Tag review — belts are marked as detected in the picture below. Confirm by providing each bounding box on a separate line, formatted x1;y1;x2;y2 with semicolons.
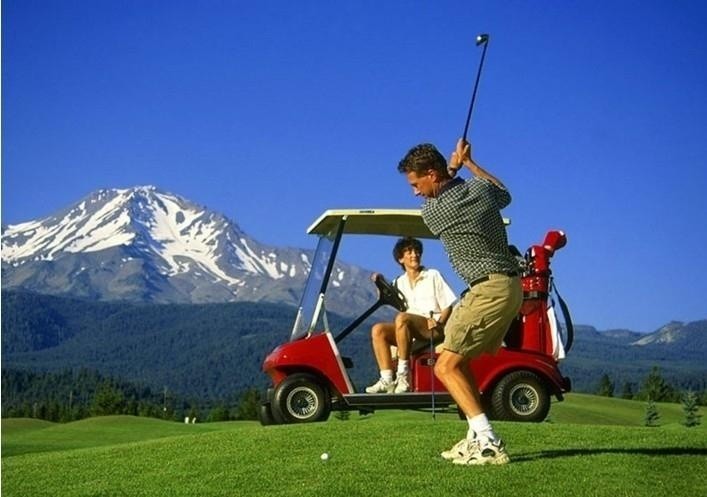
468;272;518;288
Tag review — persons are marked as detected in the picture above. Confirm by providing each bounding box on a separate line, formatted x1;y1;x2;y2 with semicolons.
364;235;458;394
396;136;523;465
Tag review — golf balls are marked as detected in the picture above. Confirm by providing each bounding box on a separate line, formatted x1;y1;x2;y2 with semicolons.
321;453;329;460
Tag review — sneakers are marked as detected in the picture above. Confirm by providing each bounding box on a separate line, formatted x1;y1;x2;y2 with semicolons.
441;438;469;460
393;371;413;394
453;438;510;466
366;377;393;394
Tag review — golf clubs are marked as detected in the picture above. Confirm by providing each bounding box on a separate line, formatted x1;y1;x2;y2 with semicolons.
462;34;489;142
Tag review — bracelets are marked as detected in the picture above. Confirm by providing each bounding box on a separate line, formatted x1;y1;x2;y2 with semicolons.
449;167;458;174
